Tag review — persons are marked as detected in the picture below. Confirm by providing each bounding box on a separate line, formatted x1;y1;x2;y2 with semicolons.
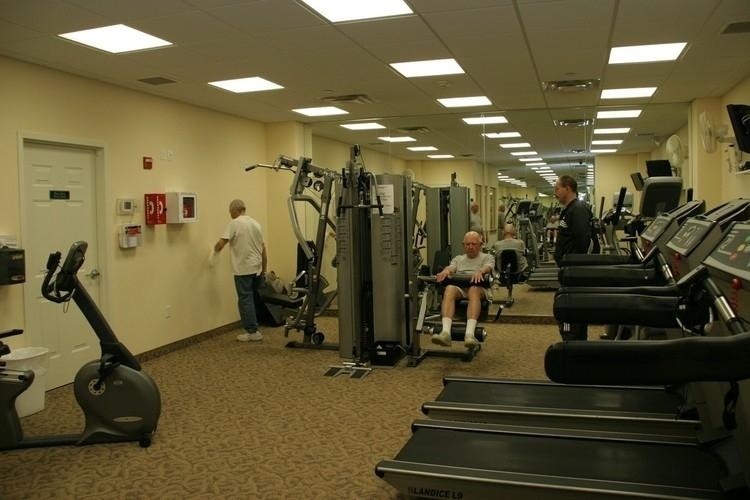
546;214;559;243
496;223;528;274
498;204;505;240
431;231;496;347
554;176;593;340
470;202;487;246
214;199;267;342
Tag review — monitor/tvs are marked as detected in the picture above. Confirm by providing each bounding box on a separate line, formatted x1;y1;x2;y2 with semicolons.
645;159;673;177
726;104;750;153
629;172;643;190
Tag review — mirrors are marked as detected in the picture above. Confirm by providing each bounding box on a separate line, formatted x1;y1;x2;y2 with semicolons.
310;102;692;317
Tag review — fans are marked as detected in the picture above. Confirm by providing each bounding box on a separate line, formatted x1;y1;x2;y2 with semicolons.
665;134;688;168
700;110;744;179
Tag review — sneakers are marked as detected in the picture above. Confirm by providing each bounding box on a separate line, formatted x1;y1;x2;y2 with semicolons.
431;330;451;346
464;334;480;348
236;331;263;342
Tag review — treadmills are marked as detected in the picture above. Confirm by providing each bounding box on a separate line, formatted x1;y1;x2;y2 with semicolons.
528;208;633;289
374;200;749;499
